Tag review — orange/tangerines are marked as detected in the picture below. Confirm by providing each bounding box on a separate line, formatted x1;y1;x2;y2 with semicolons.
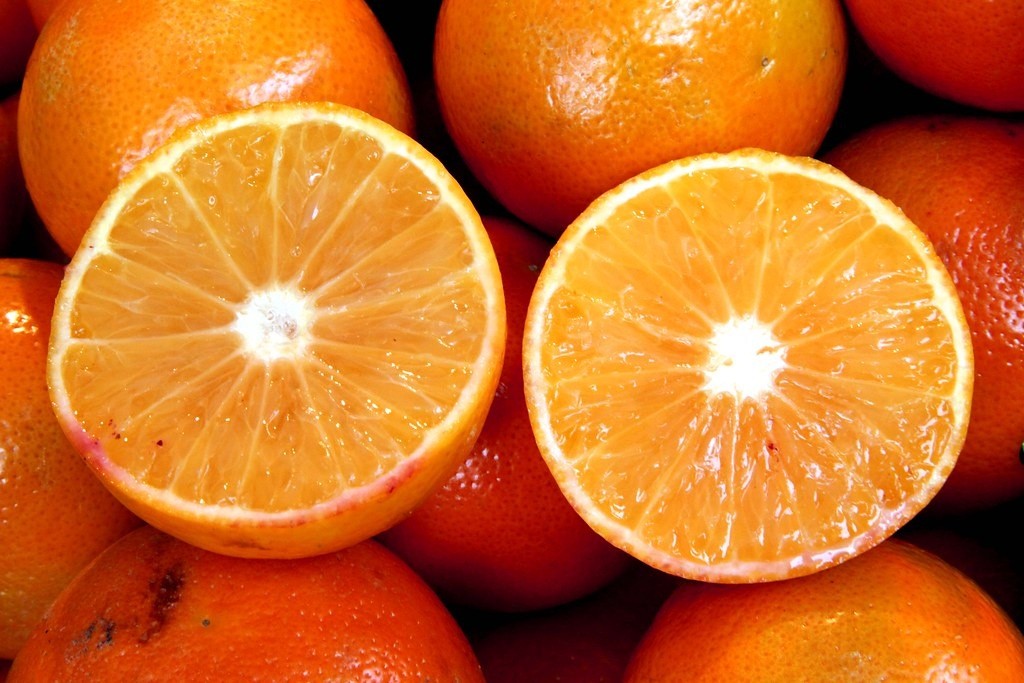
0;0;1024;683
